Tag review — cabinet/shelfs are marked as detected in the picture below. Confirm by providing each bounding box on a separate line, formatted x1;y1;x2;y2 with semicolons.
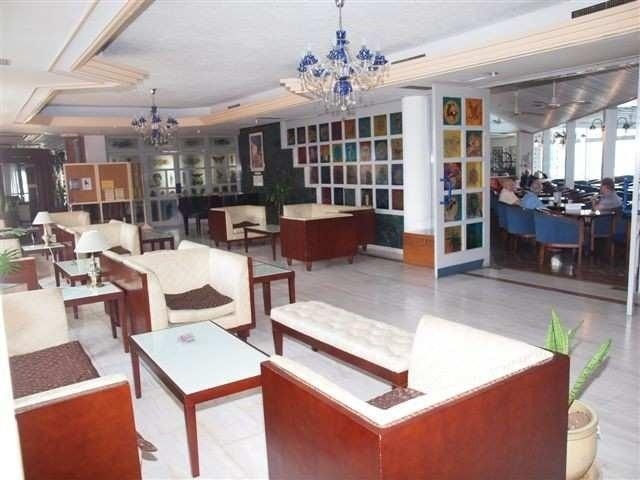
63;199;135;225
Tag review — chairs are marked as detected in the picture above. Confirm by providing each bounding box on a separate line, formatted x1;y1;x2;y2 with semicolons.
279;204;377;271
208;204;267;250
48;211;144;287
1;286;142;479
100;240;256;353
491;176;636;277
0;238;39;290
259;314;571;480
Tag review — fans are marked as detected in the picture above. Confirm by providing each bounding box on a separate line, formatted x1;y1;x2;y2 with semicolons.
532;80;592;115
495;88;545;118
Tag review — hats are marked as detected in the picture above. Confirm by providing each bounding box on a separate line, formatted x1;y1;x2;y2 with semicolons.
530;182;543;192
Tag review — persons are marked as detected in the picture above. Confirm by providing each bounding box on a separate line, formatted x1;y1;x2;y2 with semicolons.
151;173;163;187
582;178;622;256
520;176;546;209
499;176;521;207
491;177;503;197
520;168;535;186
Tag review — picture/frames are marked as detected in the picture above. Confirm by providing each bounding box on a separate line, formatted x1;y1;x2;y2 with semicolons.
249;132;265;172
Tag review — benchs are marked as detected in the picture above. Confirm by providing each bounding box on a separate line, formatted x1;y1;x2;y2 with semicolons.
270;299;416;390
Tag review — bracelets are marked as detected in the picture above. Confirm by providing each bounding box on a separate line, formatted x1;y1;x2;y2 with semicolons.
592;199;597;202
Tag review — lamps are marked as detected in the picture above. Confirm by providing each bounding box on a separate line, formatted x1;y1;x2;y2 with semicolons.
130;85;179;148
297;0;393;113
32;211;57;248
532;117;634;149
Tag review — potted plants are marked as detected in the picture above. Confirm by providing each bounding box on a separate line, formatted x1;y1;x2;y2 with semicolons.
543;308;613;480
